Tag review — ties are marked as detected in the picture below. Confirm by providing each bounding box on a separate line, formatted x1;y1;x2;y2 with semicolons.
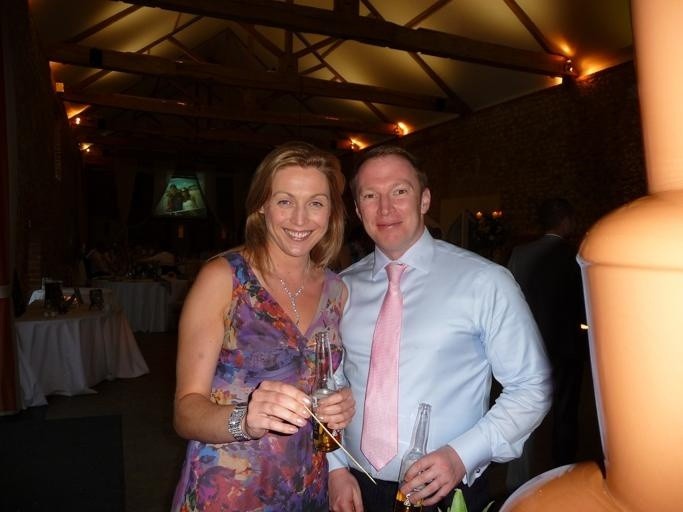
359;263;405;473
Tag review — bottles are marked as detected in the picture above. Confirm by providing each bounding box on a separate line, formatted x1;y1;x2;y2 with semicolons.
311;331;343;453
392;403;432;512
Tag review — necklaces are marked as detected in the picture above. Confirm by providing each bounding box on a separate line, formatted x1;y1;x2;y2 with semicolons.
279;278;307;328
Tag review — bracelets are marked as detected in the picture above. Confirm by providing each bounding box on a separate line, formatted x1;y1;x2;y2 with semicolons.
227;405;252;443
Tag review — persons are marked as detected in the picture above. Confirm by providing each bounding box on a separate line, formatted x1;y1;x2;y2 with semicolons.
508;195;585;467
166;184;182;212
180;187;198;210
170;143;356;512
323;145;555;511
